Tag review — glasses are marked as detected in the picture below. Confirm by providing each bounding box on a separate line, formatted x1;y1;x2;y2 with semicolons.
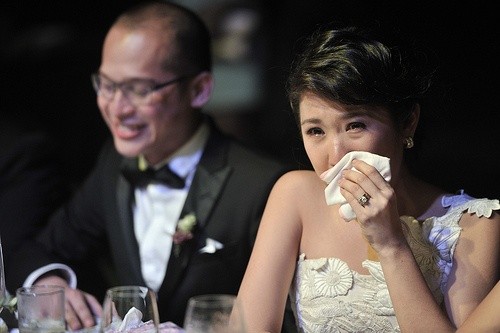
92;73;199;107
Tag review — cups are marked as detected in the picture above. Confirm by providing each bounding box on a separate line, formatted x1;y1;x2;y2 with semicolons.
97;284;159;333
183;291;246;333
15;285;66;332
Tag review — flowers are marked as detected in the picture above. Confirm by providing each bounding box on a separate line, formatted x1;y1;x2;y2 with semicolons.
172;213;197;246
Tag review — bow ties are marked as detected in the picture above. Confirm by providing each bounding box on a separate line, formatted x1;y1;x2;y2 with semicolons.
121;163;186;190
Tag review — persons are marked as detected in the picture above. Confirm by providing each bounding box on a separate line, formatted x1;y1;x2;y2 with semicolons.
1;0;297;332
229;28;500;333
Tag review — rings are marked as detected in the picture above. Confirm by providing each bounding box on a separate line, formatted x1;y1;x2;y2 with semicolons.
357;193;372;206
